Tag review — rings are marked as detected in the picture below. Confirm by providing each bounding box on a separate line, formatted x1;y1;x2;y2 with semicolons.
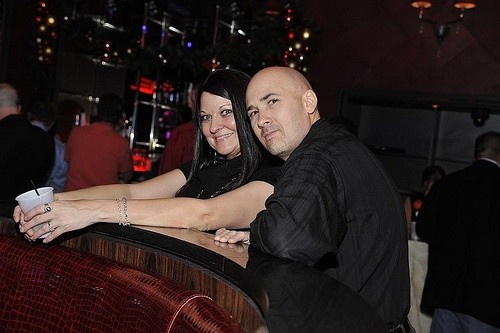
48;221;54;231
44;202;50;212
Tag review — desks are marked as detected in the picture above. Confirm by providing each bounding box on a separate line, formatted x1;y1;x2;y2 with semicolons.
407;239;432;333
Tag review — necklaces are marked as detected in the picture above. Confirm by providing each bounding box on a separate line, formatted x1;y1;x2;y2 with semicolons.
197;151;239;197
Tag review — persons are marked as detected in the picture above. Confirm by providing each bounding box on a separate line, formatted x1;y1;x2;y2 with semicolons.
13;69;288;244
405;132;500;333
0;79;199;220
214;66;410;322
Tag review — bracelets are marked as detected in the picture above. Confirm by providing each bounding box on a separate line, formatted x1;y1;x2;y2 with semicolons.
116;196;129;226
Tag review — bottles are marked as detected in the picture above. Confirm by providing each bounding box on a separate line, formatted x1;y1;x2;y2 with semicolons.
158;80;186;106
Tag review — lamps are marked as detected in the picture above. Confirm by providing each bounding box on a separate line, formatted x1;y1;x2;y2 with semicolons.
411;0;477;45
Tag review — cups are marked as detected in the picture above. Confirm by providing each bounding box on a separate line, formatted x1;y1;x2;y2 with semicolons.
14;185;55;242
